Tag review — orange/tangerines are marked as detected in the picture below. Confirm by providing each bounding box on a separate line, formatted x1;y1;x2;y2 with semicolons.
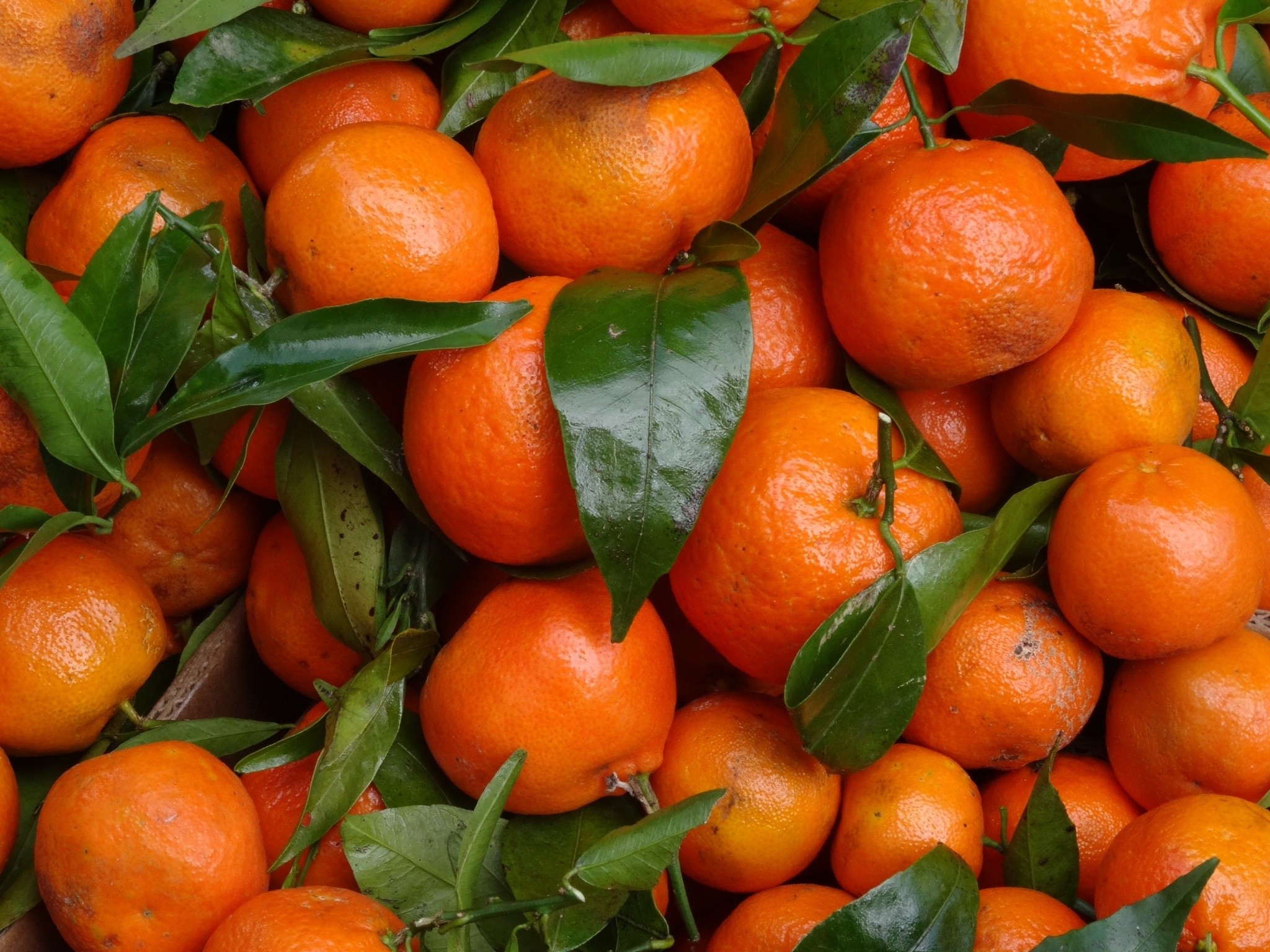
0;0;1270;952
